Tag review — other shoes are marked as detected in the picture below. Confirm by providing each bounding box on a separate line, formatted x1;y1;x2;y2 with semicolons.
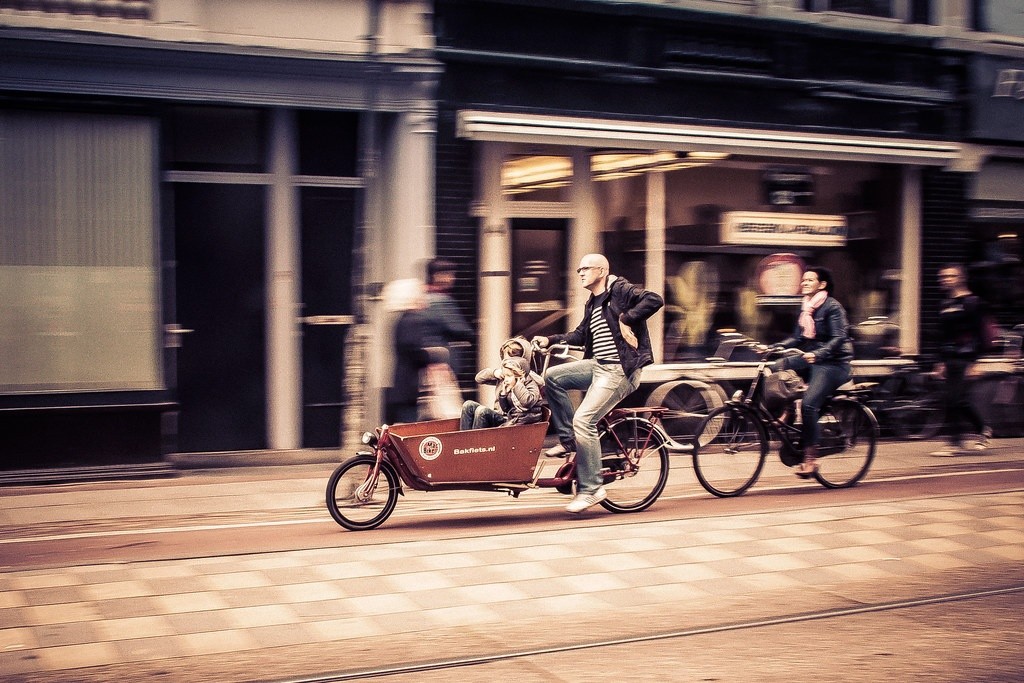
770;408;796;427
972;426;992;449
794;448;819;475
929;446;962;456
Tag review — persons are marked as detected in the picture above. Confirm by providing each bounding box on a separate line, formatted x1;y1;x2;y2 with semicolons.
930;263;1004;458
530;254;664;512
392;258;542;433
748;268;853;478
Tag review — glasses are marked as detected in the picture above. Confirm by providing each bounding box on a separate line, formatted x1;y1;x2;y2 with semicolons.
577;266;600;273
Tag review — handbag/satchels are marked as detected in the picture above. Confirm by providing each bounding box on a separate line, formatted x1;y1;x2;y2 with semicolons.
766;369;807;399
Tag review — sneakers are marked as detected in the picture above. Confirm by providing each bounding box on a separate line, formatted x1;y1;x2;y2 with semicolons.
544;442;576;456
565;487;607;513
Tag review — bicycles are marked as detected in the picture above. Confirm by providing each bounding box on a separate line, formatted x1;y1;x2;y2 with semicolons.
836;333;949;452
693;340;880;497
325;340;670;531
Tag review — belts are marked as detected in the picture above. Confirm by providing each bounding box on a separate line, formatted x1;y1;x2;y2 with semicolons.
596;359;622;364
